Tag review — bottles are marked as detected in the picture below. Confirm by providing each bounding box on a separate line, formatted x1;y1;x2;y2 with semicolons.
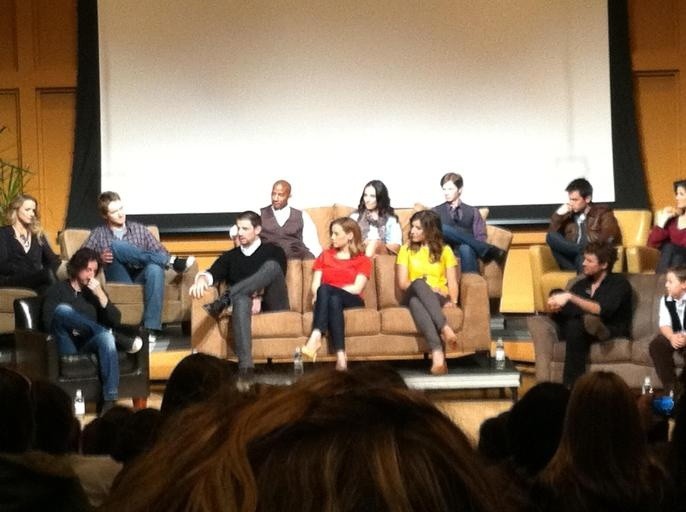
642;375;653;395
73;387;87;422
494;336;506;370
293;345;303;375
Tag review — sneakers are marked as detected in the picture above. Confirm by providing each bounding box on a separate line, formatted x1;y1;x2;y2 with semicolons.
582;314;610;343
111;328;143;353
236;367;255;379
100;401;114;417
170;255;195;273
148;330;165;343
201;293;231;320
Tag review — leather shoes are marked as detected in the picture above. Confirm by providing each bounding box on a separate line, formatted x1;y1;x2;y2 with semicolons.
334;355;348;371
430;360;448;375
442;332;458;347
480;245;506;264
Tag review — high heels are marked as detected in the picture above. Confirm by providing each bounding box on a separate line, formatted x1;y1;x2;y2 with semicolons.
301;346;317;365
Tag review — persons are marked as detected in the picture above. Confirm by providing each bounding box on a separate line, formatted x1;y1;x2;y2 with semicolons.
544;177;623;274
228;180;323;261
545;240;632;382
427;172;506;275
396;209;460;375
186;209;292;373
2;352;684;512
648;266;686;393
292;219;371;371
0;196;62;293
349;178;400;257
42;246;143;416
78;190;195;342
645;179;686;271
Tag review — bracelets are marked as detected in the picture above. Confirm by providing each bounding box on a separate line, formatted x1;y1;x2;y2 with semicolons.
447;298;459;306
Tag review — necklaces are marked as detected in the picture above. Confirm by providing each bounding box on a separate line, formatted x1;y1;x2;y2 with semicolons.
12;227;30;248
